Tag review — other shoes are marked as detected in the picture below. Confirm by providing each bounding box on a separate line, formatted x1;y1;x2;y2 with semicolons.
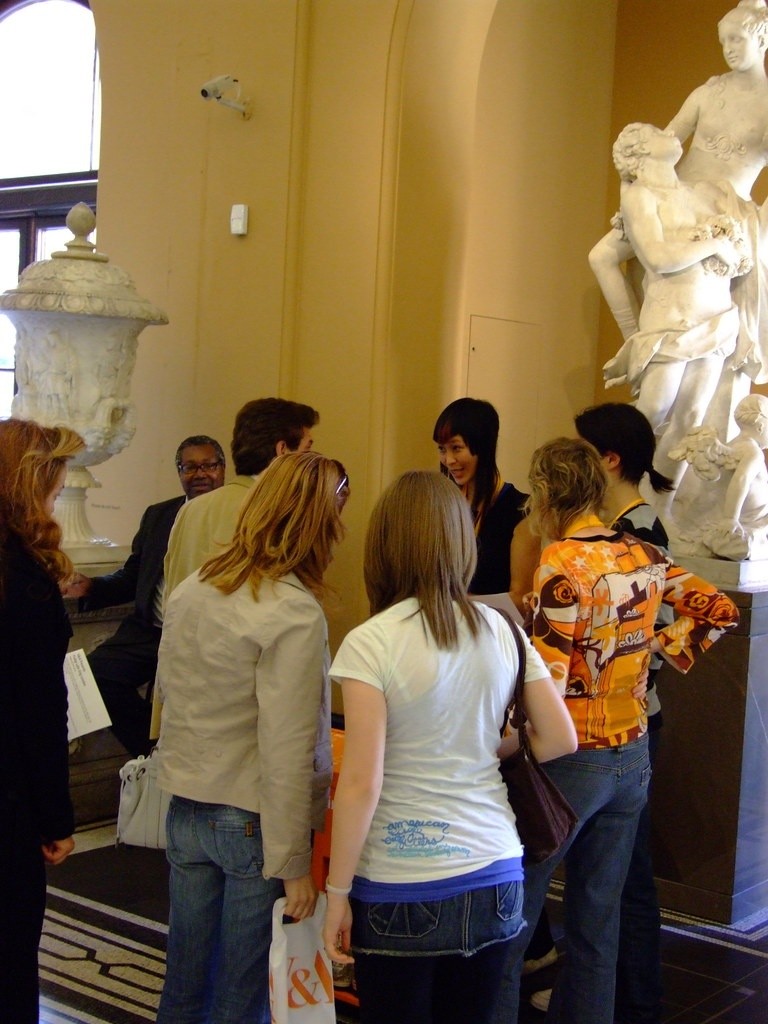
522;942;559;975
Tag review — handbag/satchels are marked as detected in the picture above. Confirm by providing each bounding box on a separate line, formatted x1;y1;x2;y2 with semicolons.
491;603;580;865
269;891;338;1024
116;749;173;848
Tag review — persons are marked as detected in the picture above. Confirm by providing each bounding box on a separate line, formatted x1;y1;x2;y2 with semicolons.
587;0;768;561
10;328;139;421
0;418;88;1024
59;397;739;1024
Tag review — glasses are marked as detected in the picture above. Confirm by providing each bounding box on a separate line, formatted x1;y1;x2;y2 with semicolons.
330;458;350;495
178;459;225;474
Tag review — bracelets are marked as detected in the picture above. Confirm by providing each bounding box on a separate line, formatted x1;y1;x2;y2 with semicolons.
325;875;352;895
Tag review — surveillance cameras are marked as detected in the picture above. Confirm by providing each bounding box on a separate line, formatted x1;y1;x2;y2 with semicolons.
201;74;233;98
